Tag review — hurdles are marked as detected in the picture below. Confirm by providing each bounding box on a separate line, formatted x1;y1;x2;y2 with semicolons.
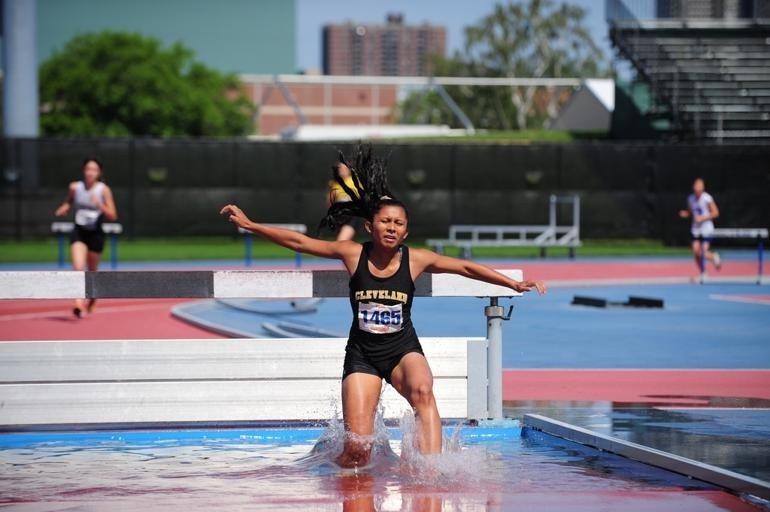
238;223;307;269
53;222;122;270
1;271;523;422
693;228;769;278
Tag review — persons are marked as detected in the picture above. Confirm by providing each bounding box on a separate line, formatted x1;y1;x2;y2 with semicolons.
326;162;365;241
220;140;547;470
52;159;123;318
342;476;442;512
678;179;722;277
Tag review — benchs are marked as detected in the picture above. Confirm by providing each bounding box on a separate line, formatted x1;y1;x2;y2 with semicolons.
621;36;770;138
